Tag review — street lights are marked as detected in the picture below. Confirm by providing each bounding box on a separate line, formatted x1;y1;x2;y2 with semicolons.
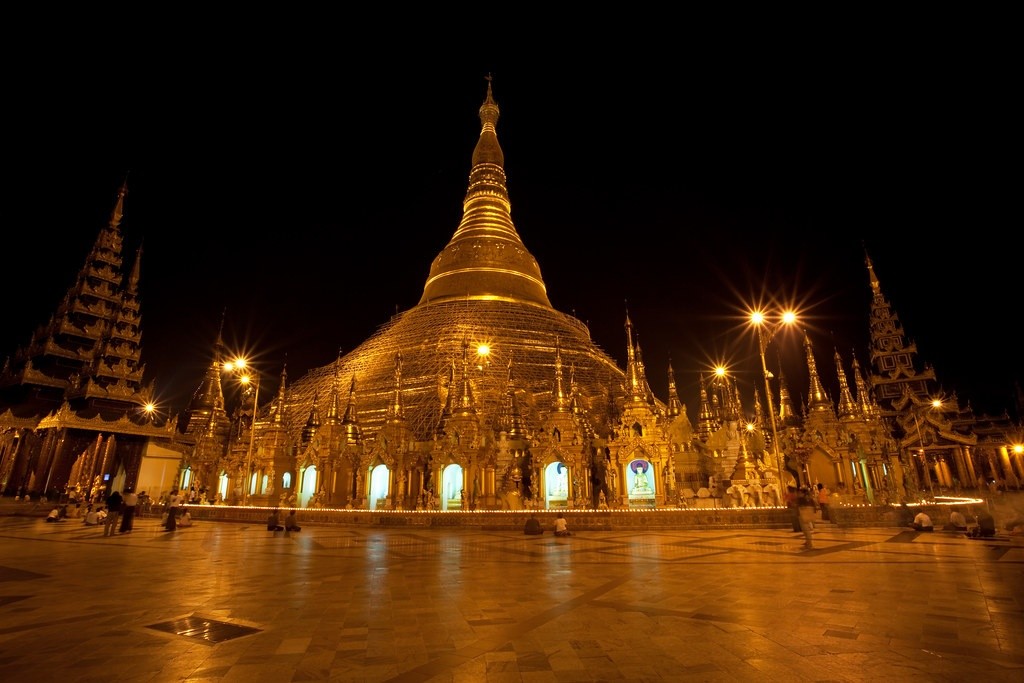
241;376;259;506
752;312;793;497
913;401;941;505
479;345;489;412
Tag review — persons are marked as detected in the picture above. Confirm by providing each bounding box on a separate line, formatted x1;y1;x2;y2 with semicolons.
908;507;933;531
968;507;995;537
285;510;301;531
189;487;196;503
552;513;568;536
942;505;967;531
46;505;70;522
71;504;82;518
15;496;20;502
24;495;30;501
102;491;122;536
40;495;47;504
786;482;829;549
83;496;109;526
524;514;544;535
120;488;137;532
69;486;80;508
267;510;284;531
160;489;192;530
